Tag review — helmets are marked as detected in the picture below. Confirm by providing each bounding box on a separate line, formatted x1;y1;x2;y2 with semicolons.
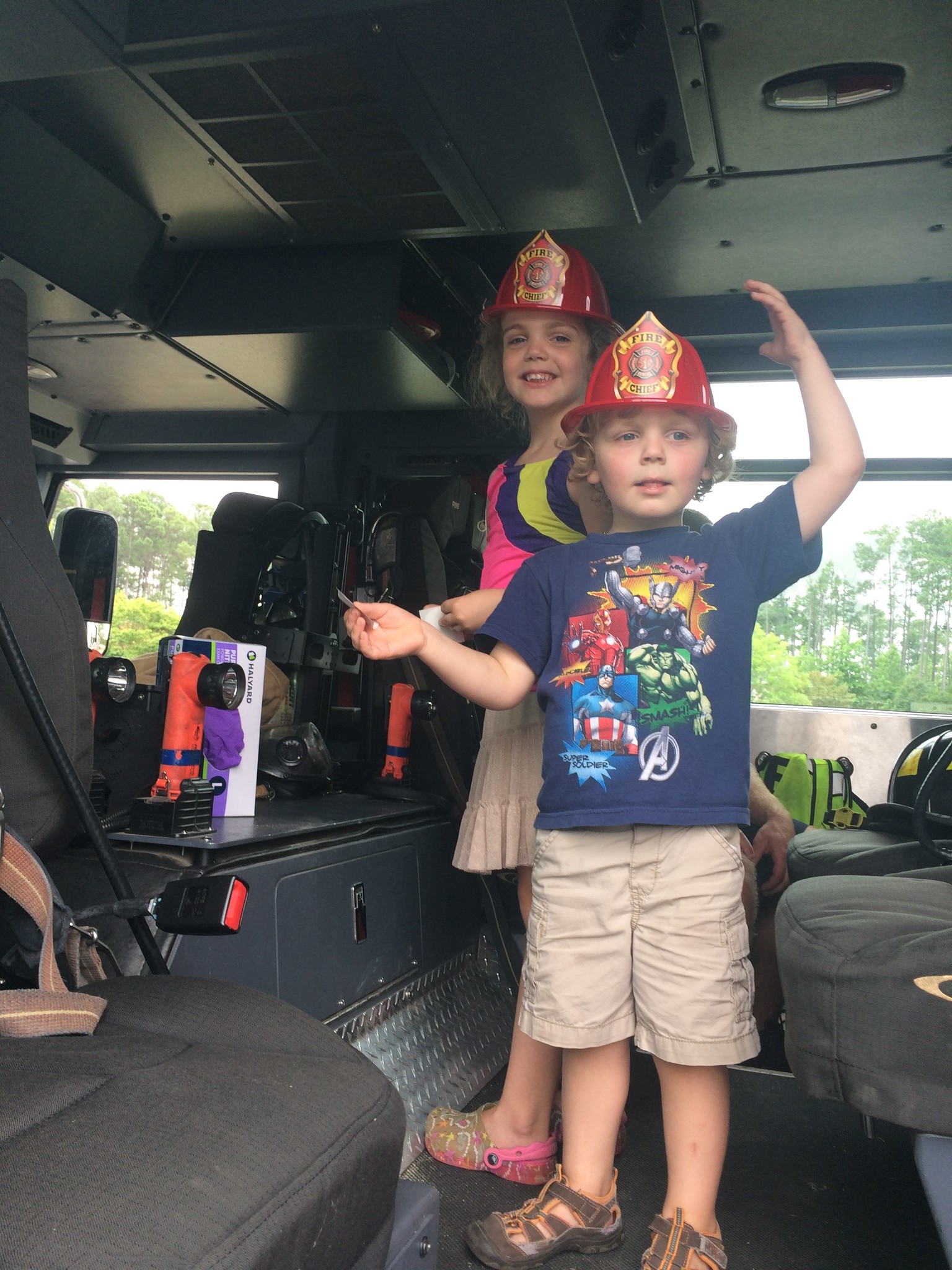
560;311;737;445
478;230;627;337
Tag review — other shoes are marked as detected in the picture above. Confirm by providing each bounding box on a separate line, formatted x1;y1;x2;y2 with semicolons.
739;1019;791;1072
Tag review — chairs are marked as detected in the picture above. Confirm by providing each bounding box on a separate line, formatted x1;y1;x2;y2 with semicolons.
0;285;406;1270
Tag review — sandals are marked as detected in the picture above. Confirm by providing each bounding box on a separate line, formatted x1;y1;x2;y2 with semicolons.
467;1164;623;1268
640;1207;728;1270
549;1087;627;1156
424;1102;559;1185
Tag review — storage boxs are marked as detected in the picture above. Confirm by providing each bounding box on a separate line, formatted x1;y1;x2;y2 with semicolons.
155;634;270;819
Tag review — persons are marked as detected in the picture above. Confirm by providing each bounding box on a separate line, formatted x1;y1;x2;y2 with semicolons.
516;759;811;1071
420;229;630;1186
341;280;866;1270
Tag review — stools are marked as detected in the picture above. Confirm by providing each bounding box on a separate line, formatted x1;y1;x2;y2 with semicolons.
785;825;951;876
771;875;952;1133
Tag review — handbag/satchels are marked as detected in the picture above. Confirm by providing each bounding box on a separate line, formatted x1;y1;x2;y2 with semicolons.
757;752;853;832
